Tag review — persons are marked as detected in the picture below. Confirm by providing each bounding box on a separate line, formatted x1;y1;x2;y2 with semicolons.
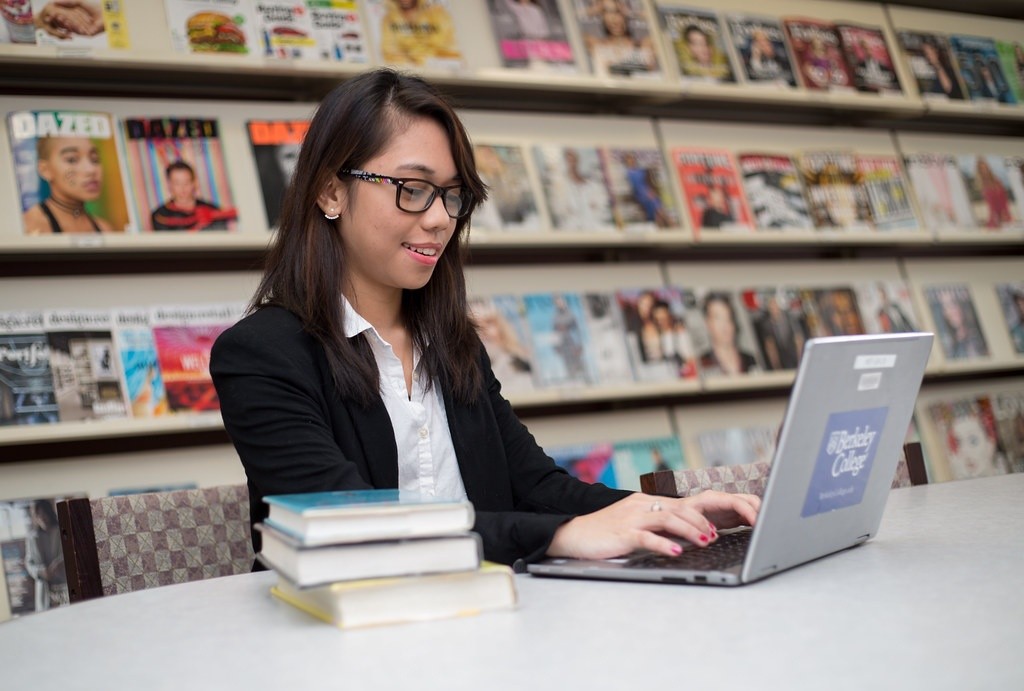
24;500;68;613
210;67;762;573
632;292;655;364
624;155;668;230
649;301;694;365
151;162;225;231
22;126;113;236
972;158;1010;229
552;150;604;228
590;0;656;78
685;19;712;64
33;0;104;39
699;292;755;376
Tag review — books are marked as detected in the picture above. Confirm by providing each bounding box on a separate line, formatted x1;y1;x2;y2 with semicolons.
0;0;1024;101
6;110;1024;235
252;488;518;631
0;283;1023;426
0;389;1024;623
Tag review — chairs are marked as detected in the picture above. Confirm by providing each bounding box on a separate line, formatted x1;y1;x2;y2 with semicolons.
56;484;256;606
640;441;929;541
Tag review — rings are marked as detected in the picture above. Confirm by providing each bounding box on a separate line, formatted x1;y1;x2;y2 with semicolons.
650;500;664;513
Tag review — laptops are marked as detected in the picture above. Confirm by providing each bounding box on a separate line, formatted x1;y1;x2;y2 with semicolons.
527;332;935;584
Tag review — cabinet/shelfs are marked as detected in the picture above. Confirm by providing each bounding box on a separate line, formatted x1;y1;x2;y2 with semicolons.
0;0;1024;625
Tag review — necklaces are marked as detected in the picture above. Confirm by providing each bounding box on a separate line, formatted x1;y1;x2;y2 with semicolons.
48;193;86;218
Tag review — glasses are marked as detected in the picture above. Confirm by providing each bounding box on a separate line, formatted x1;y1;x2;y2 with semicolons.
330;162;478;222
